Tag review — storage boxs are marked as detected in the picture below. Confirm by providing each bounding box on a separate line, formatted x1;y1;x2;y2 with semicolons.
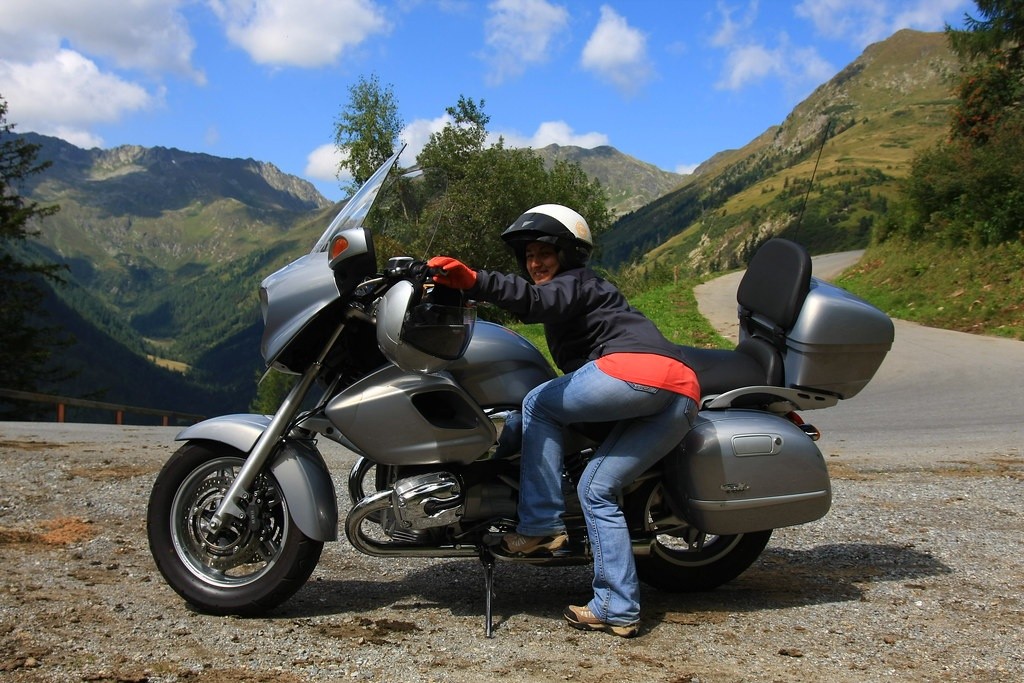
736;276;894;400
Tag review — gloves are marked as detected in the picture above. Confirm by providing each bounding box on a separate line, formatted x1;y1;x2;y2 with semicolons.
425;256;477;290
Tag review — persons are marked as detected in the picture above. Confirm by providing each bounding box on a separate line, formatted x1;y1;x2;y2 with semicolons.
427;204;703;636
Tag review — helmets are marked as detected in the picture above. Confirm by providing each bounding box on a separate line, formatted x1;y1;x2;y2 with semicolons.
502;203;593;270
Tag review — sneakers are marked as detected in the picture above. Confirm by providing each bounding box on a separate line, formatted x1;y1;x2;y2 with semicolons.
562;603;641;637
487;524;569;561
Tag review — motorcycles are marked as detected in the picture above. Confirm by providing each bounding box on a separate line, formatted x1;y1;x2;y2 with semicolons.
143;140;898;610
